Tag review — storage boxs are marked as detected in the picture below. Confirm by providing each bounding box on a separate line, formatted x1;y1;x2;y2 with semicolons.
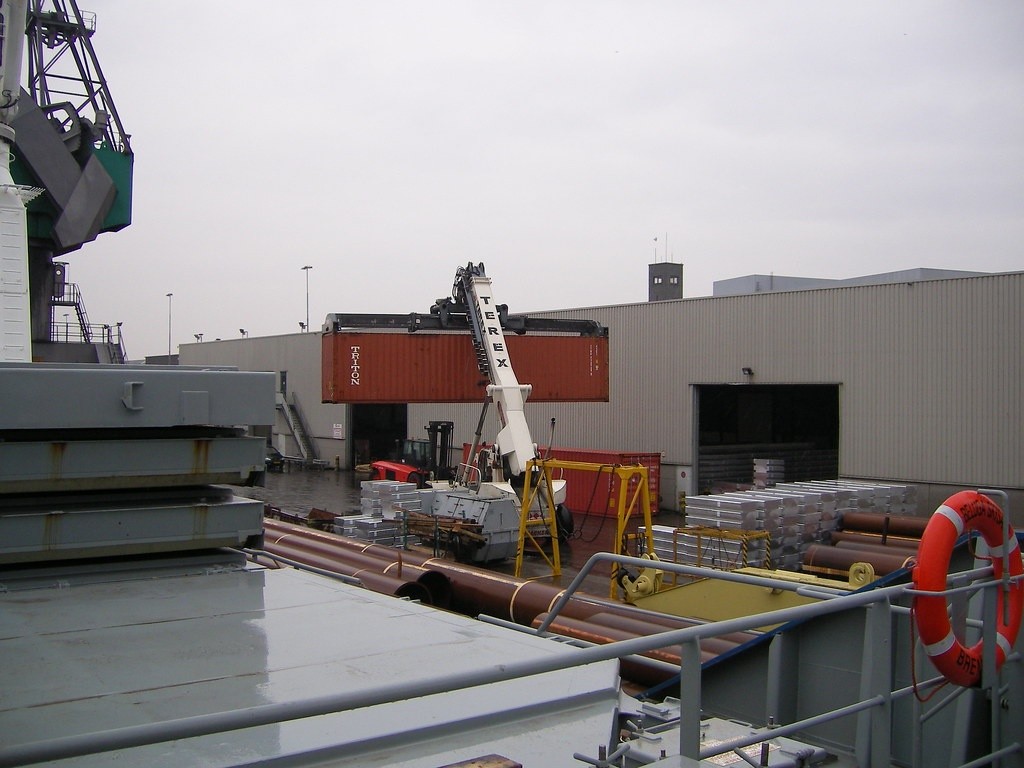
463;442;662;519
321;330;610;402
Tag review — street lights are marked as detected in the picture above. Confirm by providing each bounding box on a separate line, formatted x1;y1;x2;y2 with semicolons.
63;313;69;343
300;265;313;333
166;293;174;364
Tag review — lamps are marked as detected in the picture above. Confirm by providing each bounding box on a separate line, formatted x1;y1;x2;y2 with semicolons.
299;322;306;334
741;367;754;375
194;334;200;342
240;329;248;339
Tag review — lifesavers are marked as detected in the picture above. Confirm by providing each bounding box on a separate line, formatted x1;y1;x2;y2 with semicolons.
912;489;1024;688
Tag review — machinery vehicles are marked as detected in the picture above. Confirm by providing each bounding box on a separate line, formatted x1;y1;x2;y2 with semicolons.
424;260;574;544
368;418;454;491
